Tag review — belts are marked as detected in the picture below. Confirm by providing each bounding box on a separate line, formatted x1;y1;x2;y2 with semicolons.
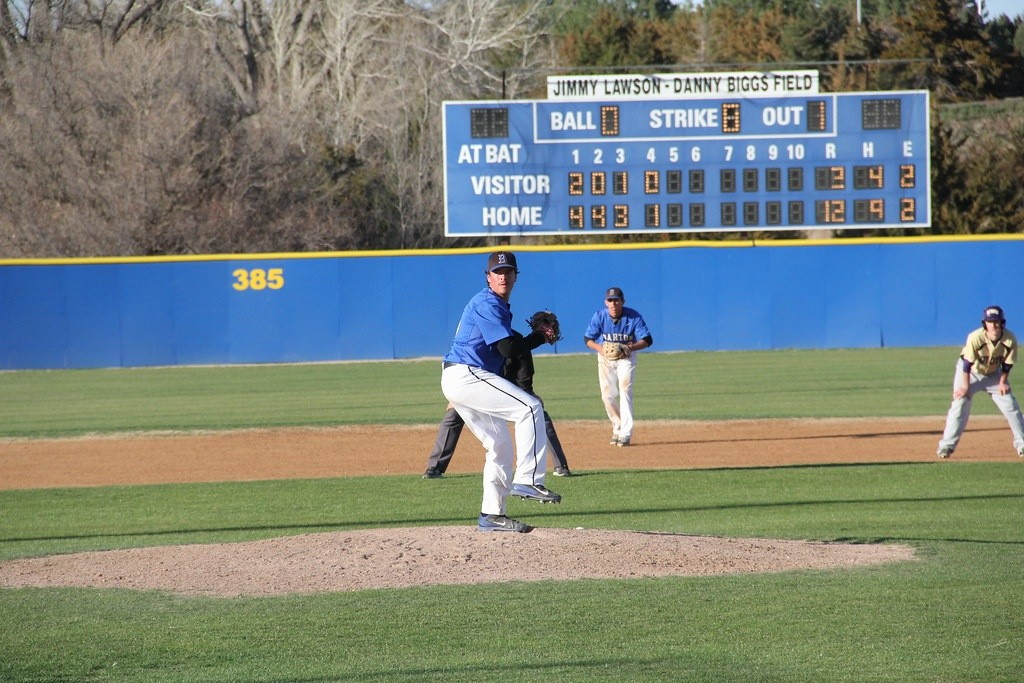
444;362;456;368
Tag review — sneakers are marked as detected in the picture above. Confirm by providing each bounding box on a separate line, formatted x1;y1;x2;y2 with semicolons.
553;466;571;477
509;483;561;504
421;467;443;478
478;513;527;532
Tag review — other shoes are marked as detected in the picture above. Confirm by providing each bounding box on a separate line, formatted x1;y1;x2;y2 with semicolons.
1019;447;1024;457
610;435;618;445
937;448;951;457
617;436;631;446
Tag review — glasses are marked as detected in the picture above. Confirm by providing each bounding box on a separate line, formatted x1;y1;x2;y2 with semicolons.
607;298;622;302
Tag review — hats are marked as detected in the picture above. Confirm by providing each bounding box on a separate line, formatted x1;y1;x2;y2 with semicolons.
606;288;624;299
488;251;517;272
984;306;1003;322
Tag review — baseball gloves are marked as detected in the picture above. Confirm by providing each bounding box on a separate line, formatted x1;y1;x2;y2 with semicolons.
602;341;631;361
525;306;564;345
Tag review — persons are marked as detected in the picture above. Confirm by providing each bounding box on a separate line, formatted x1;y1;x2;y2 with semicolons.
422;328;572;479
441;251;561;533
937;306;1024;459
585;287;653;447
